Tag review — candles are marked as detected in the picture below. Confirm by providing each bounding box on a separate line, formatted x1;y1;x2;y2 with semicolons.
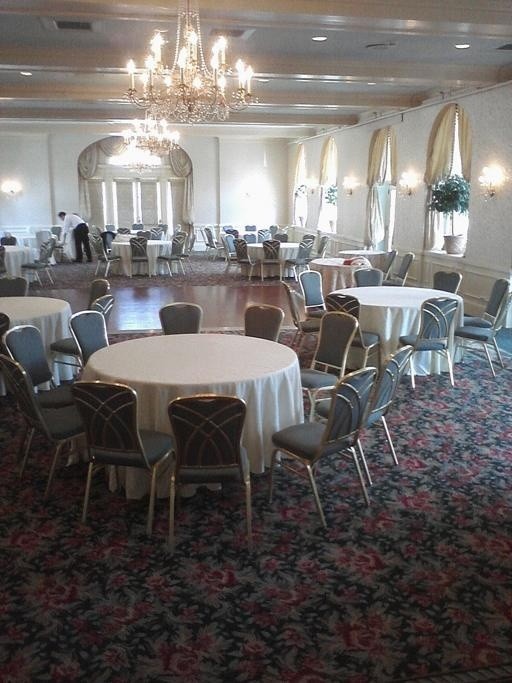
178;47;187;83
246;66;253;93
152;32;162;61
147;56;154;86
127;59;135;90
220;35;226;64
236;60;244;87
211;56;218;88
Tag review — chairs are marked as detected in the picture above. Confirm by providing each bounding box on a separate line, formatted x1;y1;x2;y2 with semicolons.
70;381;175;538
87;233;123;278
37;232;51;242
168;394;254;554
151;228;161;240
3;325;77;407
68;310;109;367
159;224;168;237
262;240;283;281
278;234;288;243
117;228;130;234
201;225;216;243
456;286;512;375
245;226;256;232
397;297;459;388
281;281;322;350
382;252;415;287
204;228;227;261
309;237;325;260
324;294;381;368
267;367;378;526
102;232;116;252
224;234;237;261
86;279;110;312
311;345;414;465
245;304;285;342
284;239;313;281
463;279;510;328
105;225;115;232
0;276;28;296
244;234;256;243
378;250;398;279
159;302;203;335
51;226;61;240
354;269;383;287
235;240;261;280
1;236;17;246
223;225;234;231
300;312;359;422
138;232;151;239
221;237;239;272
258;230;272;243
303;235;314;241
0;246;7;275
22;238;54;287
1;355;84;500
96;227;102;234
433;271;462;296
186;232;194;238
132;224;143;229
50;295;115;367
299;270;326;312
271;225;279;232
157;235;186;275
130;237;152;278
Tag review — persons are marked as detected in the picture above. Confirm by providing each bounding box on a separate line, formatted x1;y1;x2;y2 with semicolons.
58;212;93;263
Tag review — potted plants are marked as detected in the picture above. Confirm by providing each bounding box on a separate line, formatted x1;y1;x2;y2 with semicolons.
427;175;472;255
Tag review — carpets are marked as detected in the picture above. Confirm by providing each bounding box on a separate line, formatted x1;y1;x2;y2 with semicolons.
0;253;302;287
0;329;512;682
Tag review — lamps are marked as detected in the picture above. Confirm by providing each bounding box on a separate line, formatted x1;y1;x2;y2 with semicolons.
107;126;161;174
398;170;417;196
122;1;257;124
478;166;508;200
342;177;358;197
122;111;181;159
